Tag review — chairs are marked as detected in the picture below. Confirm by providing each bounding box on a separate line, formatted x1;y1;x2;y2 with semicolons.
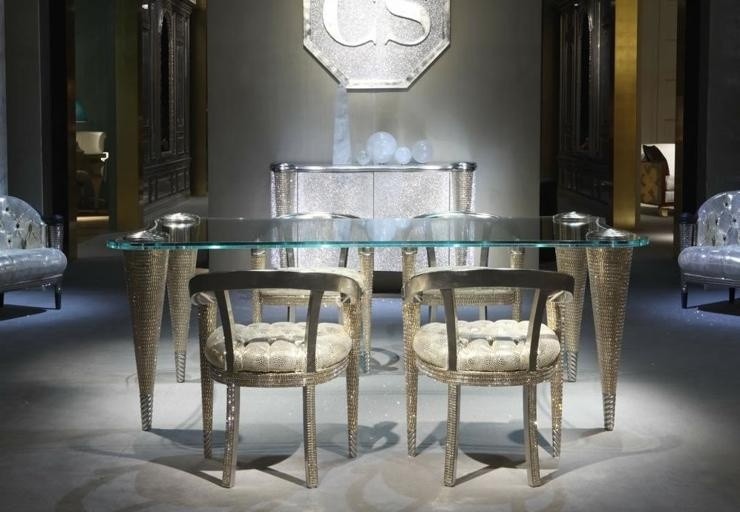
0;194;68;311
400;267;579;489
189;265;364;491
678;191;740;308
251;211;375;377
401;212;526;322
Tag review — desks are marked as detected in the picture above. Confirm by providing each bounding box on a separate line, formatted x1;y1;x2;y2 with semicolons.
106;211;650;431
